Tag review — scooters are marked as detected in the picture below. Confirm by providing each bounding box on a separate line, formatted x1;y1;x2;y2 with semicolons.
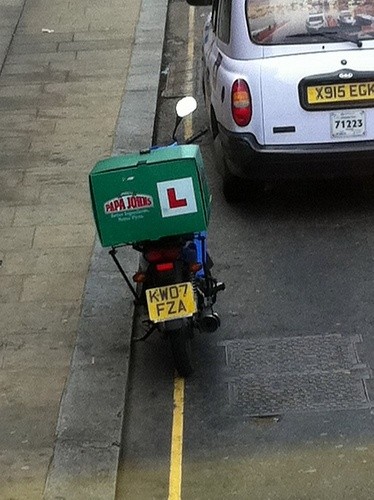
132;95;226;380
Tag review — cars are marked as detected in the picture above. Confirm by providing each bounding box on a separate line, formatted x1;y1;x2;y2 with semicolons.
186;0;374;205
